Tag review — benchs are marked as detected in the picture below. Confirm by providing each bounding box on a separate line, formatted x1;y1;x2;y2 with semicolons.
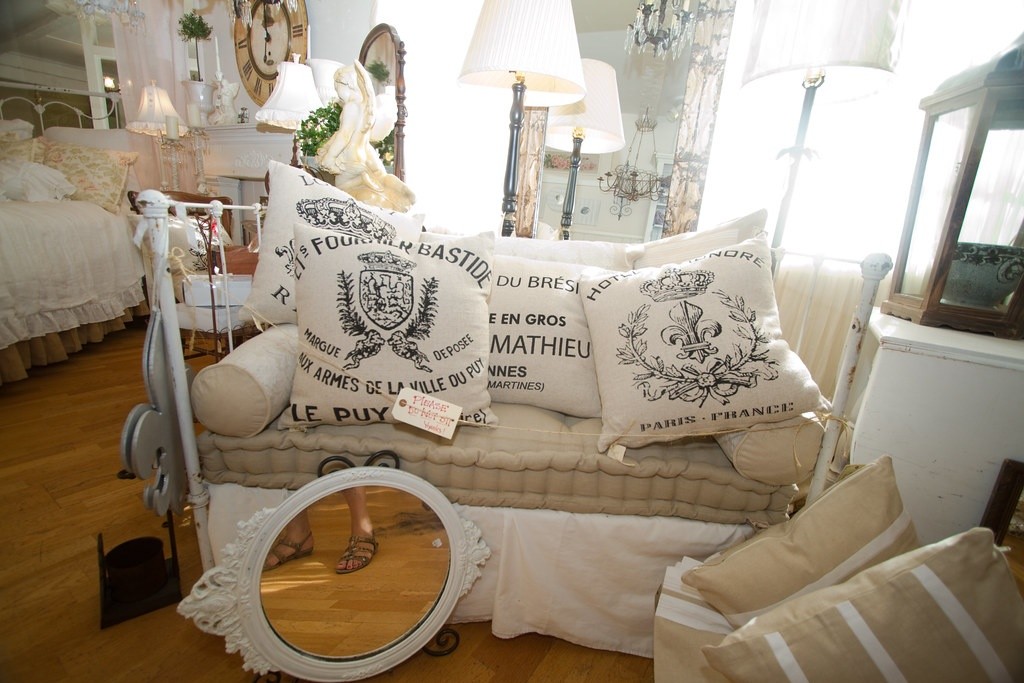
136;190;894;660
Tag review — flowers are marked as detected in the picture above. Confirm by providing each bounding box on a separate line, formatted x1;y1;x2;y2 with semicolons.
543;153;596;172
294;100;341;157
371;128;397;167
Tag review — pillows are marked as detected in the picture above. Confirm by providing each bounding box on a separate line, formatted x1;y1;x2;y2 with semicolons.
0;119;139;214
486;255;625;418
681;455;920;631
278;224;499;430
700;527;1024;683
130;214;233;303
577;233;833;453
237;160;425;327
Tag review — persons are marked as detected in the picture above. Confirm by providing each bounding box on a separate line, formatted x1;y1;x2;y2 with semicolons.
264;485;378;573
316;59;415;204
208;70;239;125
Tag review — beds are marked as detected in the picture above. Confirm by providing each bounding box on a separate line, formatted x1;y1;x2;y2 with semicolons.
0;81;151;384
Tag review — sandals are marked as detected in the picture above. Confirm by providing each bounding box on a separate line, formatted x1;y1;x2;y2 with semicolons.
262;531;314;570
336;529;379;574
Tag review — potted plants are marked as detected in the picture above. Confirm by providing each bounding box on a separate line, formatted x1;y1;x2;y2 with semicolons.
176;9;218;127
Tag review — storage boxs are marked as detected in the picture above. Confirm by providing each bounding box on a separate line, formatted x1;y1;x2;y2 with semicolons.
182;274;252;306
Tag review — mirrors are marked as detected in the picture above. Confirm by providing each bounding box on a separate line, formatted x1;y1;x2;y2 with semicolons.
178;450;490;683
980;458;1023;597
513;0;736;244
358;23;406;183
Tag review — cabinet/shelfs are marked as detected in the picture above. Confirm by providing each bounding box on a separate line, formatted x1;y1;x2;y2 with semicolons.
642;152;674;242
844;307;1023;548
241;220;257;246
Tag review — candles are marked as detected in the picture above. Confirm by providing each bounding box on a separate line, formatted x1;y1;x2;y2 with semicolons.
185;104;201;127
215;37;220;73
165;116;180;140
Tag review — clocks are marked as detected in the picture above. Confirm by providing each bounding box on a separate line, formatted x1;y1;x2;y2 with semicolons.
233;0;311;108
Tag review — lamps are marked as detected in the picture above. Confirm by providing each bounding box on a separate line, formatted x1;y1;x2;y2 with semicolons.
597;105;673;222
623;0;697;61
741;0;907;276
103;76;116;92
73;0;147;36
254;52;328;167
543;58;625;239
126;80;189;191
457;0;587;237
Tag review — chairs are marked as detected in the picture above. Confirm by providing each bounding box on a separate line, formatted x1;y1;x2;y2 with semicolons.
128;190;259;359
